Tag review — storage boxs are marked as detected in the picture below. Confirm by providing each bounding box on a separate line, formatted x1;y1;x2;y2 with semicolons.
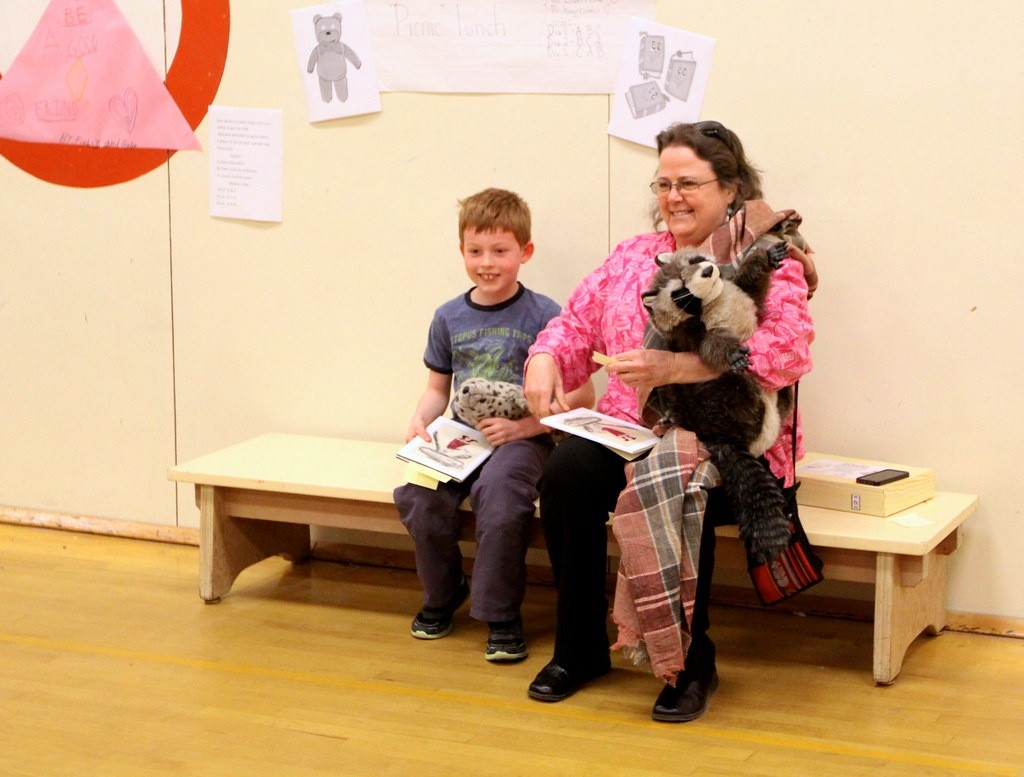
795;450;934;519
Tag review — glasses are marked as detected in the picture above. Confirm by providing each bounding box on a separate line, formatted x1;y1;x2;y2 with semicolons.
648;174;727;191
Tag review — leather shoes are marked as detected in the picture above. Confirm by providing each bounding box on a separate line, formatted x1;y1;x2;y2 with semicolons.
652;670;719;722
529;656;610;701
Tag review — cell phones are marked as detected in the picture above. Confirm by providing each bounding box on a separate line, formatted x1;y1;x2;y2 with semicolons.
856;469;910;487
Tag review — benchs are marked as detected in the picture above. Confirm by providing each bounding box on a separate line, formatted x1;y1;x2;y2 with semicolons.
168;434;979;685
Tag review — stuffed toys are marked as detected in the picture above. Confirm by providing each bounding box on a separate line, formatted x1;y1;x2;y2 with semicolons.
641;239;790;559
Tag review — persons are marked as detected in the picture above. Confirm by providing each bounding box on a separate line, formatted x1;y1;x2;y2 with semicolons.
523;119;816;723
393;188;562;663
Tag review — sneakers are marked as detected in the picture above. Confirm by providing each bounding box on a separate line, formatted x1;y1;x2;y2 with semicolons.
484;615;529;660
410;576;470;638
